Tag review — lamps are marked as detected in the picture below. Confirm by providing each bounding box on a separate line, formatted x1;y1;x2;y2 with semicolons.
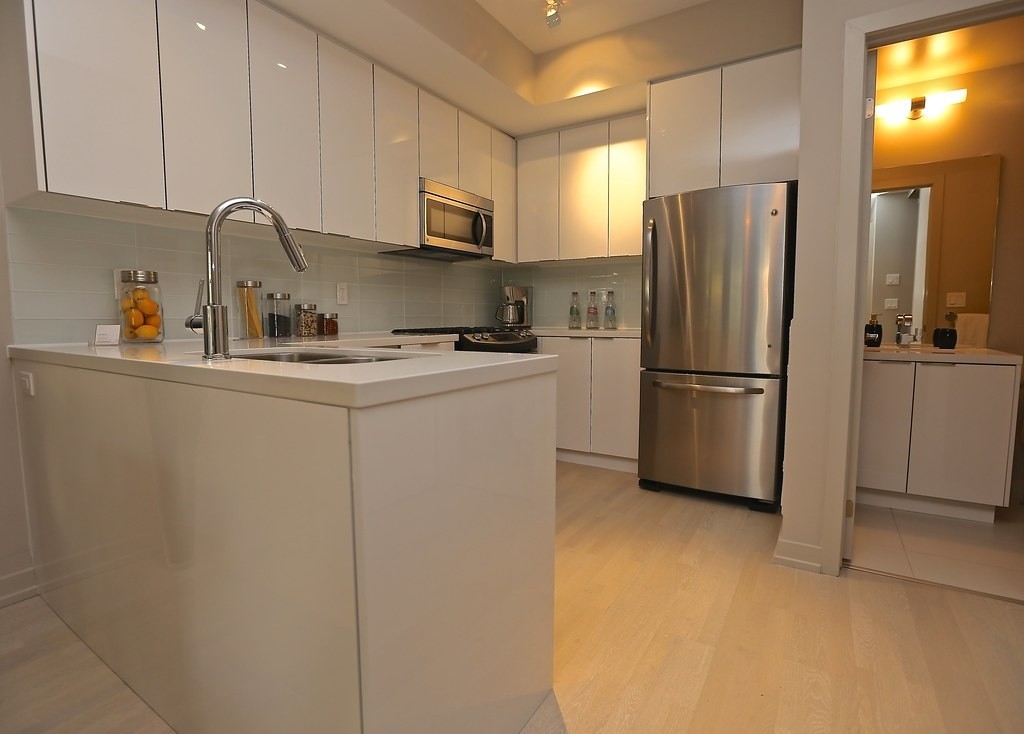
874;86;968;120
543;0;564;27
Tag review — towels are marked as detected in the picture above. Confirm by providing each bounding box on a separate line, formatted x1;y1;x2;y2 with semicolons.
954;313;989;348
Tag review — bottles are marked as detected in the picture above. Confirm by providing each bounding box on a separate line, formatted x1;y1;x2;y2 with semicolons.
118;270;164;343
604;291;617;331
237;280;337;339
586;292;599;329
569;292;582;330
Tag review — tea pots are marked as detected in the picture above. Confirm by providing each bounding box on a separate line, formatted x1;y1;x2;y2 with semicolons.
495;304;520;323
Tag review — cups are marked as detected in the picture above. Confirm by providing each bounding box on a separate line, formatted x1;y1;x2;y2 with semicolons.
940;329;957;349
933;328;945;347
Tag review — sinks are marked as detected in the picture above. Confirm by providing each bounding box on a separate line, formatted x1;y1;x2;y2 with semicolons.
866;348;957;354
229;352;373;363
302;356;407;364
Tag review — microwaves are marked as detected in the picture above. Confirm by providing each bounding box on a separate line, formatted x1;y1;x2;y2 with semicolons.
378;178;494;263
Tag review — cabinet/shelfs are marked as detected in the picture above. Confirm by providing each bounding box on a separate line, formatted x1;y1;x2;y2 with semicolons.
856;346;1022;522
0;0;801;264
537;336;640;475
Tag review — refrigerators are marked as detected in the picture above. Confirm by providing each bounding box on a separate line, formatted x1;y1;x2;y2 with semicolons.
638;183;796;514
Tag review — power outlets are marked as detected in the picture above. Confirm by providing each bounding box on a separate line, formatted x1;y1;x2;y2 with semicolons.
337;283;348;304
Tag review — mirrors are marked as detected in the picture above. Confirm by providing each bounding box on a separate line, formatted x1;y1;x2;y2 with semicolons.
870;154;1002;347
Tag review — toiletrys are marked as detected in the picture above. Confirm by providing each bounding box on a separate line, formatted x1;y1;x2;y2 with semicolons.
864;312;884;348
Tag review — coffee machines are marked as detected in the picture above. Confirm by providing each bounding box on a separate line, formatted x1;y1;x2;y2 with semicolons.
500;286;532;327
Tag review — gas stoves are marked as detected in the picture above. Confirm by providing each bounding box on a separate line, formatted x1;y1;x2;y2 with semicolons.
391;327;537;353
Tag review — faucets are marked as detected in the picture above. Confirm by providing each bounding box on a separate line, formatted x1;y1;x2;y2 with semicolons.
895;314;904;345
899;314;918;348
183;196;309;360
